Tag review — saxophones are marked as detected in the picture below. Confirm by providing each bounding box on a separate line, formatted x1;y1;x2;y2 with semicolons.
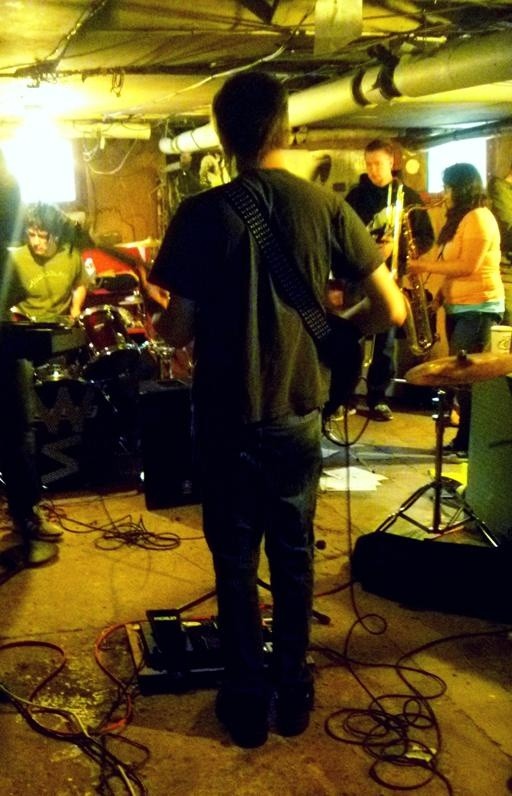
402;203;448;358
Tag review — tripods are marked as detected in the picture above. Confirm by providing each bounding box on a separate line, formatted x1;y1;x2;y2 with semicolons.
376;390;503;550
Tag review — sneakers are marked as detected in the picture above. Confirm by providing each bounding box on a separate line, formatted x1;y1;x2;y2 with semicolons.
15;509;64;539
328;404;395;421
443;442;468;460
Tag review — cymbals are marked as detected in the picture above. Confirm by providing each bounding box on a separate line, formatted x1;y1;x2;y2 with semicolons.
404;349;512;386
113;237;162;248
118;294;143;304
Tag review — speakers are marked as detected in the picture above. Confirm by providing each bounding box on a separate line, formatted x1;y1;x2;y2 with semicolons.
142;387;204;509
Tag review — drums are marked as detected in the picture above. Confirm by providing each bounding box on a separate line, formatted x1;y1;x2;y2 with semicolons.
77;307;142;379
32;363;119;488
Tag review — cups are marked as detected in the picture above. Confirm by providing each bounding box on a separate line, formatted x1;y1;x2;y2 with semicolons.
489;324;512;354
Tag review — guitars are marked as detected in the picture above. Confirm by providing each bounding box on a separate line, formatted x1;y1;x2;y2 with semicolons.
24;199;365;411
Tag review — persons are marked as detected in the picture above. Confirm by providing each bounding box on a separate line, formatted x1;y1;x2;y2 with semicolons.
489;170;512;327
6;199;92;324
405;161;505;458
1;148;65;539
145;73;411;749
329;137;435;422
